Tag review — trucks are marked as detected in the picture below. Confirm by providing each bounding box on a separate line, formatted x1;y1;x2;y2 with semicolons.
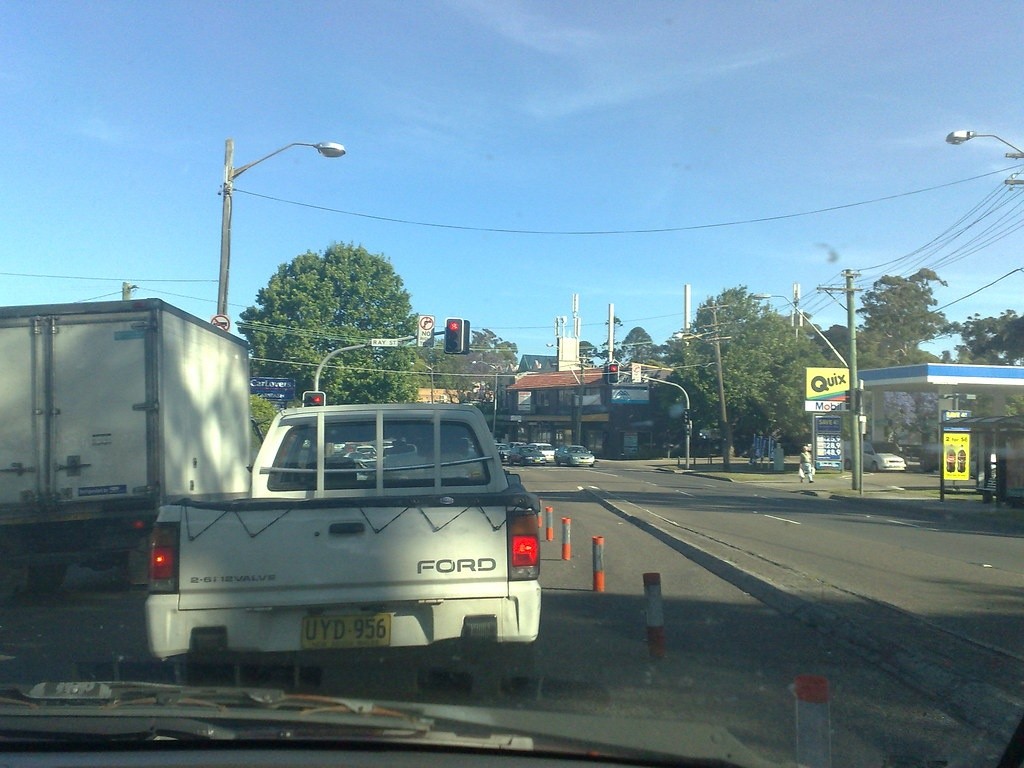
0;296;252;593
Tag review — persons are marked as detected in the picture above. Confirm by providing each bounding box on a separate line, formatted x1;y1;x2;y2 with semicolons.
800;446;814;483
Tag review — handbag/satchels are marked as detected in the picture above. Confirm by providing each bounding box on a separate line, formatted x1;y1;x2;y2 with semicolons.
799;468;805;478
811;467;815;475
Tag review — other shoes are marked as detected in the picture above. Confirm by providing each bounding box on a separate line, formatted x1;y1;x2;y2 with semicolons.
809;481;814;483
801;479;804;483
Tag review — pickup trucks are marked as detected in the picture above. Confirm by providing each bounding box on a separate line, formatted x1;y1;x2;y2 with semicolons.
143;400;544;689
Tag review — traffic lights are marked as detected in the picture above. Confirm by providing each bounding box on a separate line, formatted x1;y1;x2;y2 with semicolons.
444;317;464;355
607;363;619;385
301;391;326;407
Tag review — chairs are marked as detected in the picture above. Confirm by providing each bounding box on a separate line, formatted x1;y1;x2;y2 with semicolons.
307;457;357;487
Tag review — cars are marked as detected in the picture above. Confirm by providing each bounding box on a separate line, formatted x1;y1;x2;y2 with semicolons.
734;435;777;458
494;441;556;467
554;445;596;467
864;446;908;473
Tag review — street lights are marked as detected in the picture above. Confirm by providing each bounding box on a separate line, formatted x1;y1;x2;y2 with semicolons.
547;343;585;445
673;332;731;469
750;292;864;491
216;136;346;320
409;361;434;404
471;360;498;435
